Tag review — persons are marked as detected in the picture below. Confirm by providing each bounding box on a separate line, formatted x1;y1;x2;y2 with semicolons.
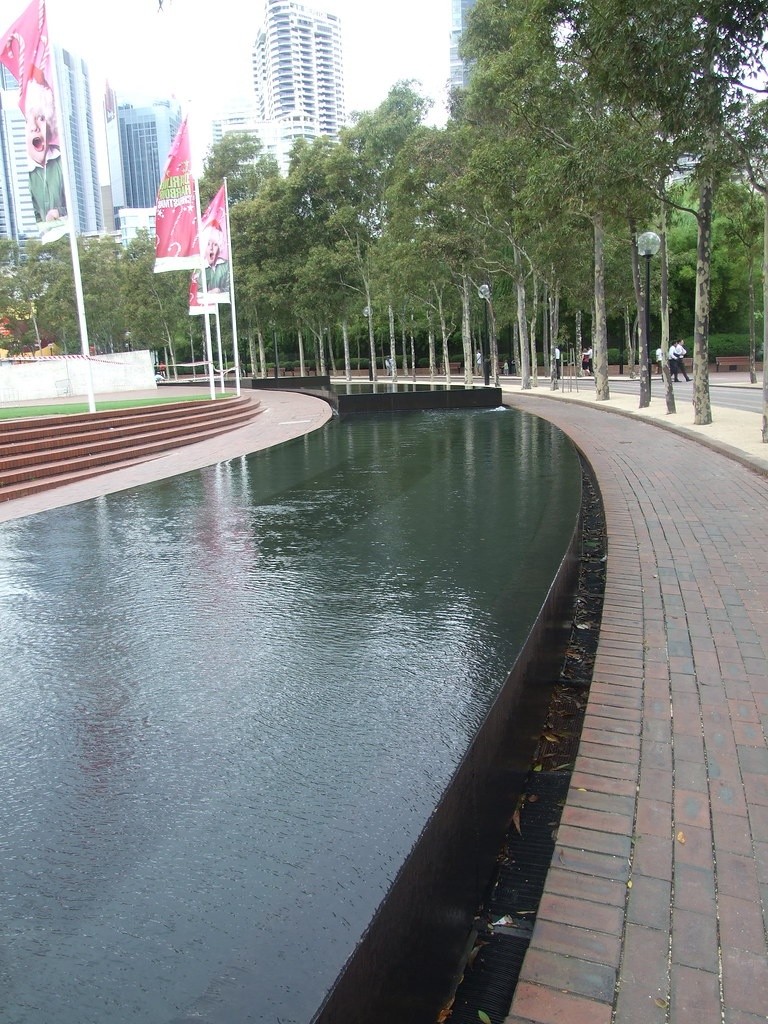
476;351;510;377
22;79;67;223
385;356;393;376
553;347;595;379
159;361;165;377
656;339;693;382
194;222;229;295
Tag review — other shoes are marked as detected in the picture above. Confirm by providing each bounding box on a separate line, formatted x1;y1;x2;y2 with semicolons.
686;379;693;382
675;380;682;382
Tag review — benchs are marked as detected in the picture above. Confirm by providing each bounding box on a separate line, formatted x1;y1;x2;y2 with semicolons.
315;366;329;376
267;368;285;376
716;356;750;373
498;362;504;374
437;362;462;374
683;358;693;373
291;366;310;376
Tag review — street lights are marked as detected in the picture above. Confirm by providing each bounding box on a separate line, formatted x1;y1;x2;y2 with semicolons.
477;284;490;387
362;306;374;381
636;231;661;396
